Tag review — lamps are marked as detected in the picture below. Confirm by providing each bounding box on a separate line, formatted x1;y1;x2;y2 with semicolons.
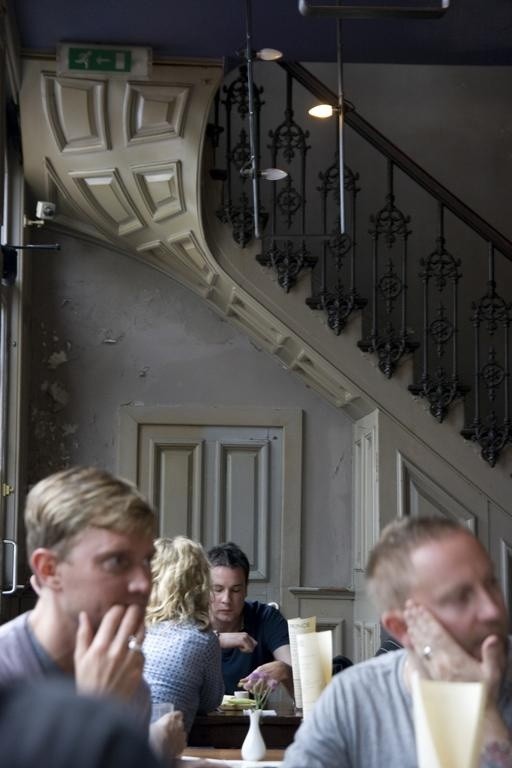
235;0;346;240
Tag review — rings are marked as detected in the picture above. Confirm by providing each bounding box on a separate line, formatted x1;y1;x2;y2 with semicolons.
421;646;433;658
128;633;142;651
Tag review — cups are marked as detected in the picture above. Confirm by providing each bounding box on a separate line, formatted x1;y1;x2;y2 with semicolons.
234;691;249;699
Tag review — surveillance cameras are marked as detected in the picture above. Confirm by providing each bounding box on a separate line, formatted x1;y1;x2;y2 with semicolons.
36;200;57;221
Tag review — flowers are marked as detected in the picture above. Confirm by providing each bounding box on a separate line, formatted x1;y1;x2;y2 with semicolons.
243;672;279;710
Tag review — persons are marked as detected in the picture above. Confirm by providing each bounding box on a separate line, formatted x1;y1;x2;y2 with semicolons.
135;534;227;737
0;463;153;745
1;673;188;767
204;542;293;700
283;511;511;767
